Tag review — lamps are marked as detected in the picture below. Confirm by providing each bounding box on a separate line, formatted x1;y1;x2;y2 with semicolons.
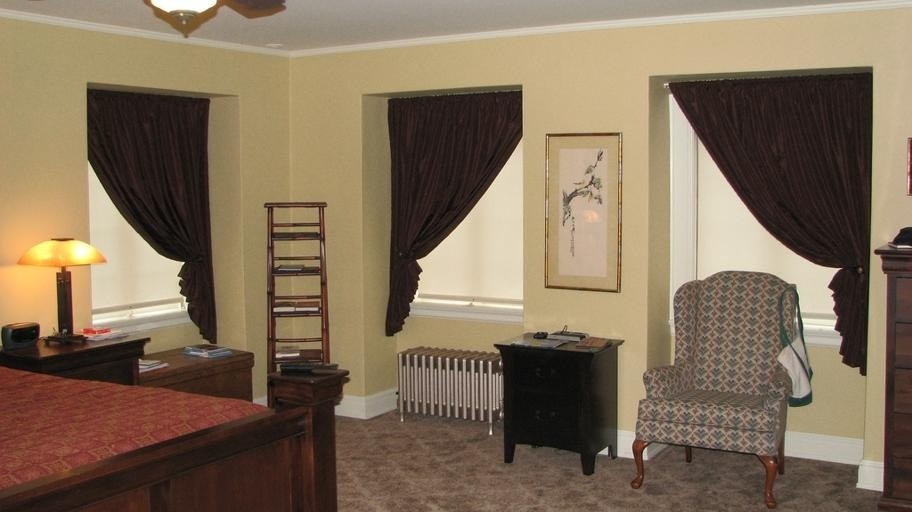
18;237;108;349
144;0;219;25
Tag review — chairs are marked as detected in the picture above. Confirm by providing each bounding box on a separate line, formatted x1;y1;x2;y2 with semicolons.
630;269;798;509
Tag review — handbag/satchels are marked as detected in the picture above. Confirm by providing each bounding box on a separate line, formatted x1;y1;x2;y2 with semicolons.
776;286;814;407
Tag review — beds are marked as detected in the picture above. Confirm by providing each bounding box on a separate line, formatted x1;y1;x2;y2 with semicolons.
0;359;350;511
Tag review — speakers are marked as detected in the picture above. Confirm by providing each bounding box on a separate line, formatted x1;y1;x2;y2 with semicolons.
1;321;40;349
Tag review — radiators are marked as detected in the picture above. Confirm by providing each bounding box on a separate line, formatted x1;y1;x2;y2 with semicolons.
396;345;504;434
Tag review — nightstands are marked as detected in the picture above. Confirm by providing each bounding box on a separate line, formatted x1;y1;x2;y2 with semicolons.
1;334;151;387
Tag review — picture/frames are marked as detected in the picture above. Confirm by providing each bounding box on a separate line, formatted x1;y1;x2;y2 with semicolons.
542;131;625;295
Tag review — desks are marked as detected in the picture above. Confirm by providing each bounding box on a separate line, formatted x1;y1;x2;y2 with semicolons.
493;330;628;476
139;343;256;404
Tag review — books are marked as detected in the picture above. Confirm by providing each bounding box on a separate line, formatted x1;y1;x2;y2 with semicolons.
185;344;231;357
278;264;303;271
297;302;320;311
138;359;169;374
547;331;584;341
275;345;300;358
575;337;611;350
273;301;295;311
278;364;312;372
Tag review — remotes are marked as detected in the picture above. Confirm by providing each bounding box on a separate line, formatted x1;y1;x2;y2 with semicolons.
277;363;339;372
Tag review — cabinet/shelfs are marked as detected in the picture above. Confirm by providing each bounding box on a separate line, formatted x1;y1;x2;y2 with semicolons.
873;238;912;512
263;200;331;407
